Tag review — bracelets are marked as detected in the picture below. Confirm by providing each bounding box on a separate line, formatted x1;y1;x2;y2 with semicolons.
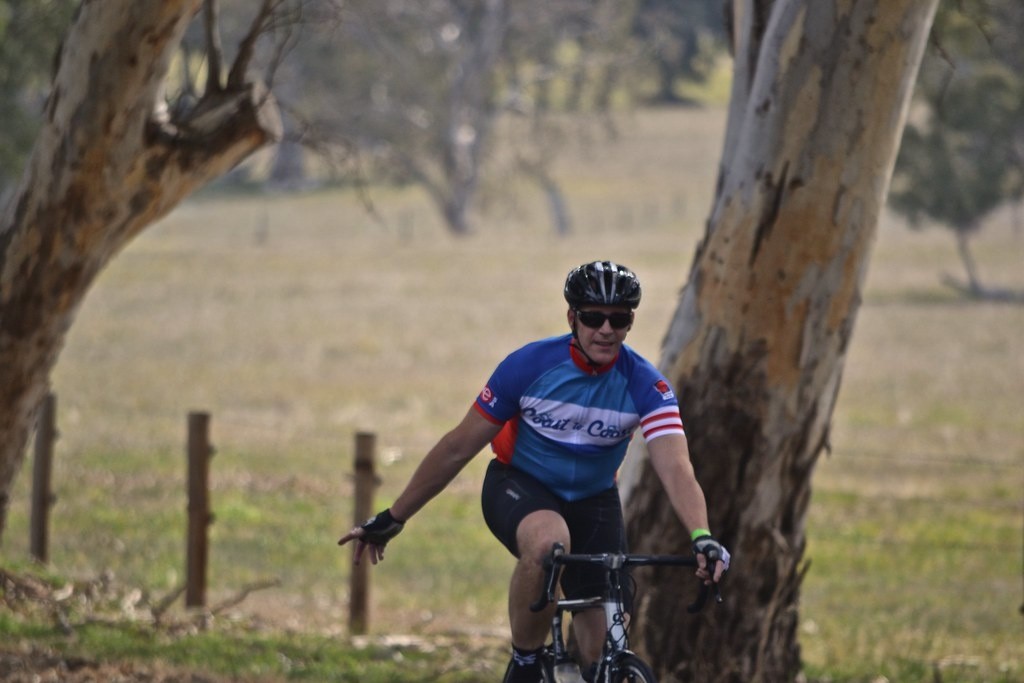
691;529;711;541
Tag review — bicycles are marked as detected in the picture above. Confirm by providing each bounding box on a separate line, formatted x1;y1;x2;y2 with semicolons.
528;540;732;683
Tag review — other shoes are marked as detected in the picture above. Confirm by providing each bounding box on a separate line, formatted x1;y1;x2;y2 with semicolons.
502;644;545;683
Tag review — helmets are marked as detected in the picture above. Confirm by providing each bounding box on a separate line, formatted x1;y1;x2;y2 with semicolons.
564;261;642;309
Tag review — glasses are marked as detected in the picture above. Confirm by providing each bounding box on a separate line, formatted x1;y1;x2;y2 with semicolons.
572;305;633;331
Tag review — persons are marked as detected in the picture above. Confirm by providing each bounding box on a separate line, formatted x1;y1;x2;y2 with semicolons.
338;262;731;683
